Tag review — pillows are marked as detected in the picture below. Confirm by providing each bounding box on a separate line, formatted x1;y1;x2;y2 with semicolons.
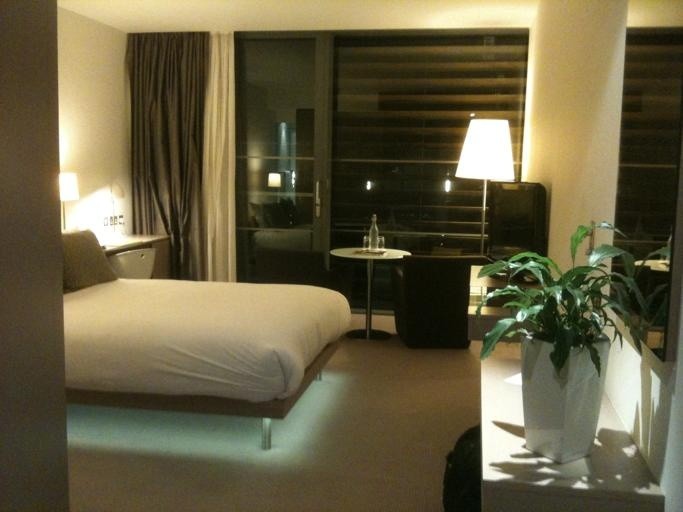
62;229;118;291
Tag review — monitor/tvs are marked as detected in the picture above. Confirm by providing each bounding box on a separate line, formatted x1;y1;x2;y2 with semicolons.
486;182;545;281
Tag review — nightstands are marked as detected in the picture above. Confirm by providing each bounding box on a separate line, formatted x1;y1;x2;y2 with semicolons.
96;234;170;278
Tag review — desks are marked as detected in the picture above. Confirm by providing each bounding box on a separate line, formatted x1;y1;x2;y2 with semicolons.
330;247;412;340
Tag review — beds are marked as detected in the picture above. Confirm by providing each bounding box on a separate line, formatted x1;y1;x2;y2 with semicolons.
62;277;351;449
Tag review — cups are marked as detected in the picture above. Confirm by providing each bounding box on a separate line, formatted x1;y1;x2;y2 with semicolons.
363;236;386;253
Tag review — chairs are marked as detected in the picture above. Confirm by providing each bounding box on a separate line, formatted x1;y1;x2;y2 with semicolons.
393;255;472;348
110;247;157;278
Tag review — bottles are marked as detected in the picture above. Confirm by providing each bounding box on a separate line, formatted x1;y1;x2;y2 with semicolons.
369;214;379;253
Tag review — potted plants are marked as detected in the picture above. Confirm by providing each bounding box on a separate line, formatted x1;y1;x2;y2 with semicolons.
478;221;641;461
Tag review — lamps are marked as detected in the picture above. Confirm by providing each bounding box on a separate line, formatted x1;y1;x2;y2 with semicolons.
454;117;517;255
